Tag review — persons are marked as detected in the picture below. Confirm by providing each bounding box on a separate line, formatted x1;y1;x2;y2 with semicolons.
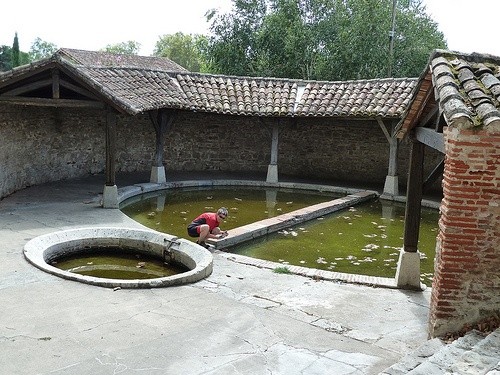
187;207;228;249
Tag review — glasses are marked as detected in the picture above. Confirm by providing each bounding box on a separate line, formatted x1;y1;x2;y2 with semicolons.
219;215;225;220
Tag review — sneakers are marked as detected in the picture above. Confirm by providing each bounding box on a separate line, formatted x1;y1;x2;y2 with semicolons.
196;241;211;249
